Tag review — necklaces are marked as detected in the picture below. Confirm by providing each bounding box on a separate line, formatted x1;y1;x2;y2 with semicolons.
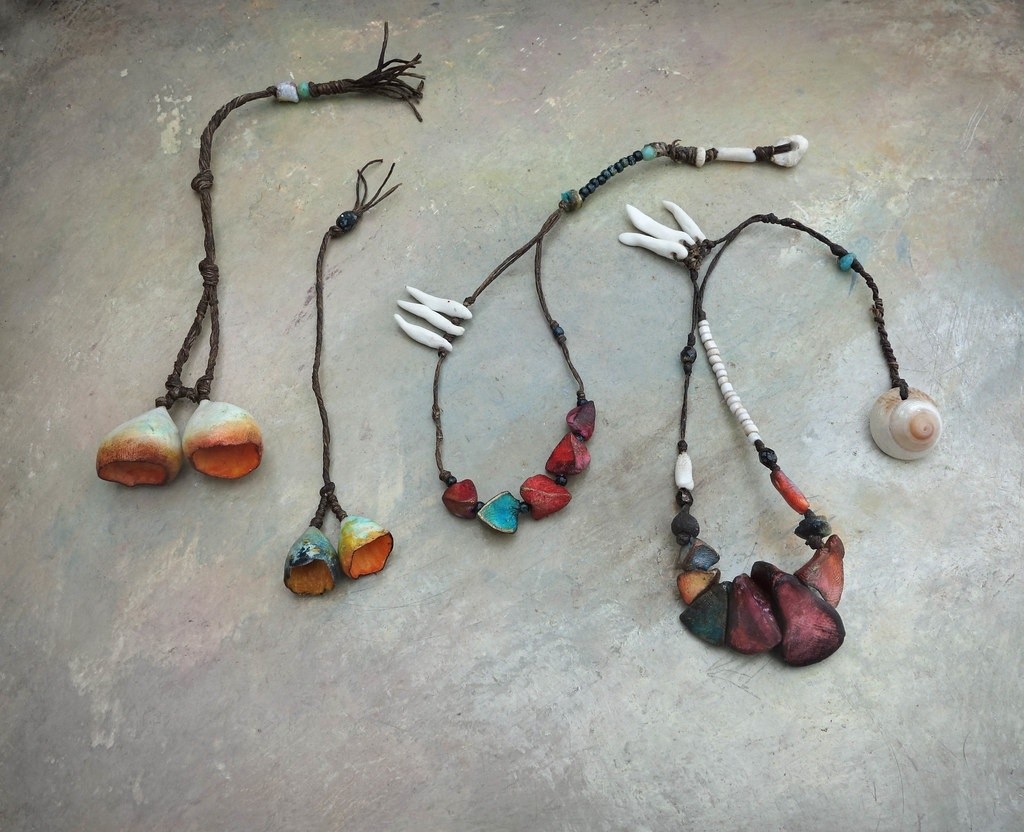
392;135;945;666
